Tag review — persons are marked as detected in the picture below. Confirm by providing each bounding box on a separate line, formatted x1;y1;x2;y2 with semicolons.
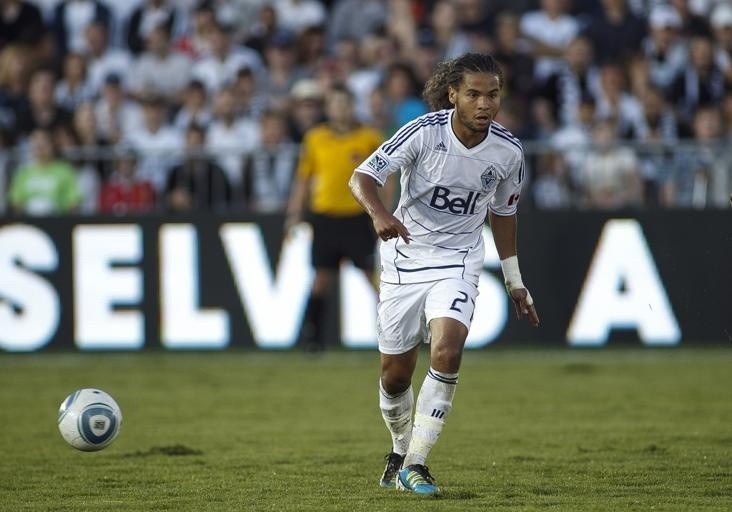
347;52;540;497
280;85;393;356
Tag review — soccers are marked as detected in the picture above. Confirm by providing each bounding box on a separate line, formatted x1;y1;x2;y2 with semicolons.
56;386;122;452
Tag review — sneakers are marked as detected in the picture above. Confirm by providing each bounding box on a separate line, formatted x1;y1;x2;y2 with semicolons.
374;444;448;498
